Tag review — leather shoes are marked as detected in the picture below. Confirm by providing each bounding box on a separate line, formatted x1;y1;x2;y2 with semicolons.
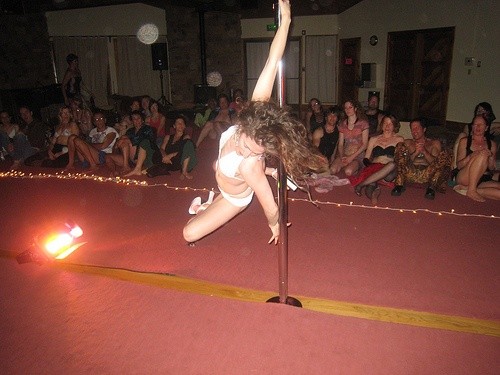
425;183;435;200
391;185;405;195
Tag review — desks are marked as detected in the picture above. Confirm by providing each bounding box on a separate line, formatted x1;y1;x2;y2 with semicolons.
353;85;378;108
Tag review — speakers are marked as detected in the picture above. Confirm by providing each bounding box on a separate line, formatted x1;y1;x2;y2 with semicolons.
151;42;168;71
194;84;218;104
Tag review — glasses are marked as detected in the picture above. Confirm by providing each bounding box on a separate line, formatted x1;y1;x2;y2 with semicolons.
95;117;105;122
477;109;488;112
311;102;319;107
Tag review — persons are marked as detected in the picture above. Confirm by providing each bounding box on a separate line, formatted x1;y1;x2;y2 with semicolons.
191;90;247;149
183;0;291;243
0;53;196;179
304;94;500;205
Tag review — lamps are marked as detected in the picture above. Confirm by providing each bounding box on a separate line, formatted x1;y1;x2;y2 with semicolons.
16;219;85;265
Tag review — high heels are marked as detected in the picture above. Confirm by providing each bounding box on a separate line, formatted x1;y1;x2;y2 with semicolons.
272;168;297;192
188;191;215;215
